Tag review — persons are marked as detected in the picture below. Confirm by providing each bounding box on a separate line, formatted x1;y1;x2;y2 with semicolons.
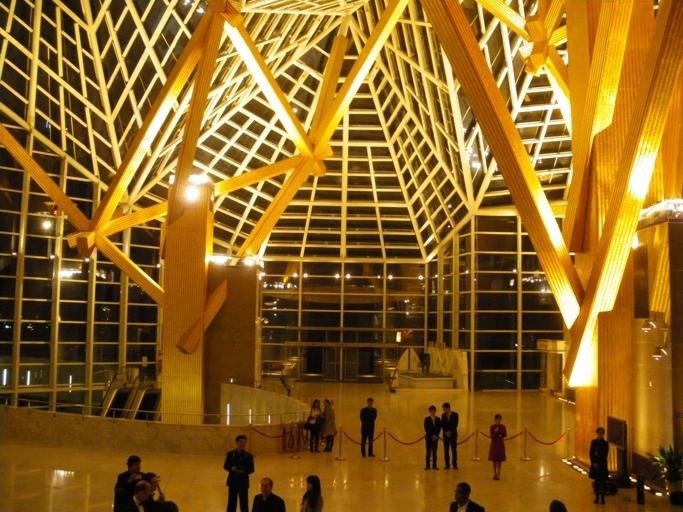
441;403;458;469
360;398;377;457
549;500;566;511
114;455;179;512
251;478;286;512
450;482;484;512
301;476;323;511
320;400;337;452
487;414;506;479
423;405;441;470
589;428;609;504
224;434;254;512
307;399;323;452
